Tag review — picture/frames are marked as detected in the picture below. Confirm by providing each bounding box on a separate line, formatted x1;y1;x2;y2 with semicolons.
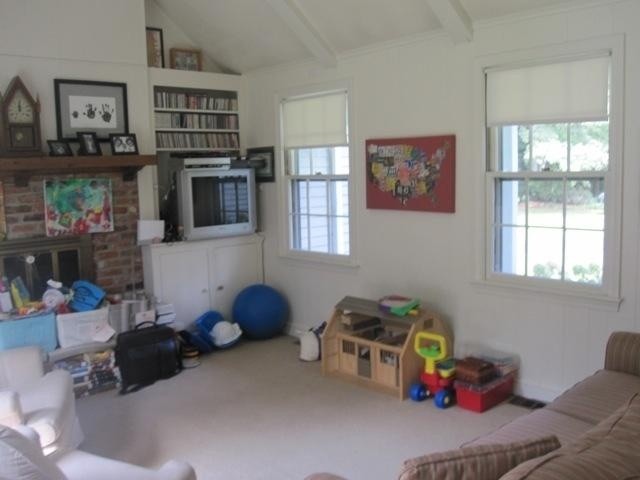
245;146;275;183
47;78;139;155
146;26;203;72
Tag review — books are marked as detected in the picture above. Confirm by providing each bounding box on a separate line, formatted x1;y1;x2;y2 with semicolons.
53;352;121;399
155;91;238;149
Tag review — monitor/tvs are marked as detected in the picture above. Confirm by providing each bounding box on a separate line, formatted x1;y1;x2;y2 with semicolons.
175;168;258;241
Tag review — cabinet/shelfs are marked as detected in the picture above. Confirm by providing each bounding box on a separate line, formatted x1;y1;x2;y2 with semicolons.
144;243;264;332
152;83;240;152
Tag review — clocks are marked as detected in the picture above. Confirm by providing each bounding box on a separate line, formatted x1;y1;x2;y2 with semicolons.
1;74;44;156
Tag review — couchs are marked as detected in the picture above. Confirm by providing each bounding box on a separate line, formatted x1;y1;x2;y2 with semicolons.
304;331;640;480
0;344;197;479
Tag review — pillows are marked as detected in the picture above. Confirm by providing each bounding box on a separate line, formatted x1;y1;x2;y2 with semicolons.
395;435;560;480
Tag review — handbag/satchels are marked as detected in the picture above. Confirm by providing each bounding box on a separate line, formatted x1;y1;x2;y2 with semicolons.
114;321;183;394
